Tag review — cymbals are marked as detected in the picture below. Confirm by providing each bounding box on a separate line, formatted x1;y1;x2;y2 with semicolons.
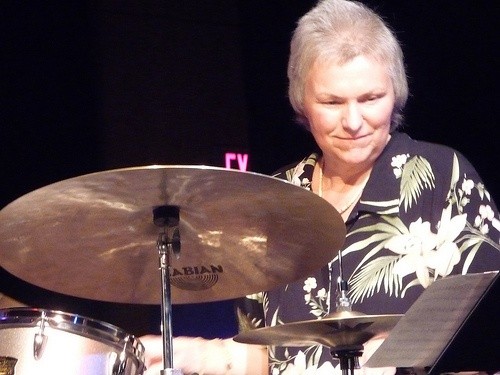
228;308;405;348
0;164;347;305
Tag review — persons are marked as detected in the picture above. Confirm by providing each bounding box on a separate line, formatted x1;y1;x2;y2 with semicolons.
231;0;500;375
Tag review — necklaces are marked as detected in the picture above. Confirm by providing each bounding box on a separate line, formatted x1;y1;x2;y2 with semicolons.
319;153;370;214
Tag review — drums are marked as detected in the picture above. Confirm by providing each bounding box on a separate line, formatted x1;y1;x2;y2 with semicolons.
0;307;147;375
